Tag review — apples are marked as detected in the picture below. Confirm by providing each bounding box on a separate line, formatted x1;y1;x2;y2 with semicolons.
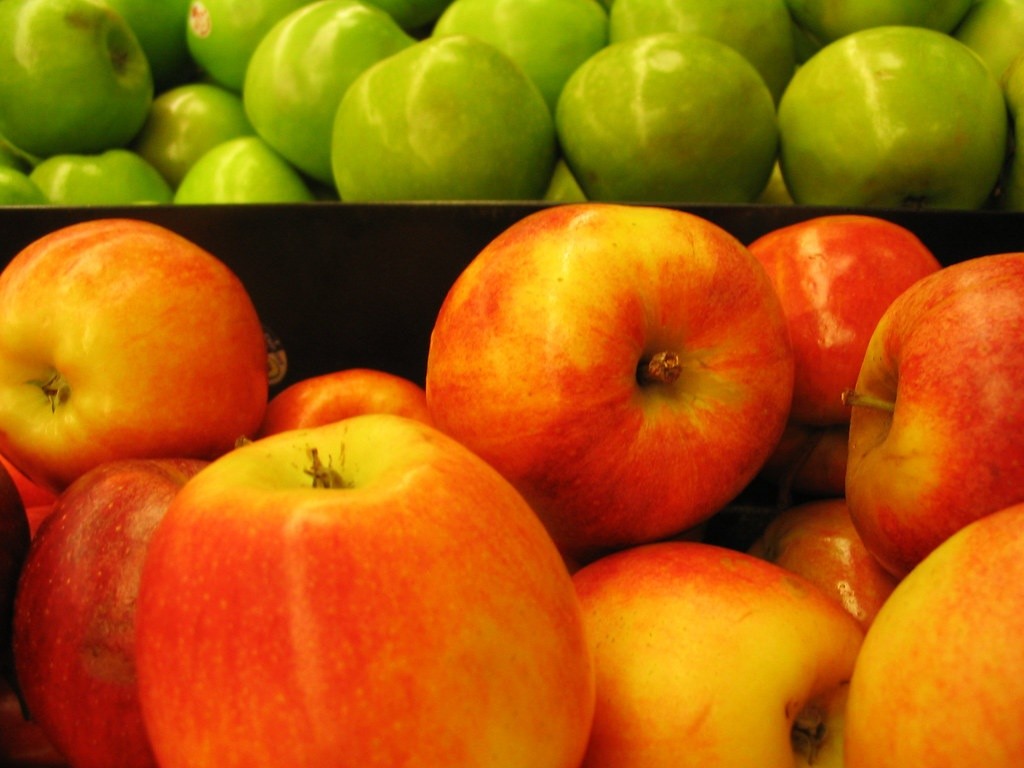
0;200;1024;768
0;1;1024;209
